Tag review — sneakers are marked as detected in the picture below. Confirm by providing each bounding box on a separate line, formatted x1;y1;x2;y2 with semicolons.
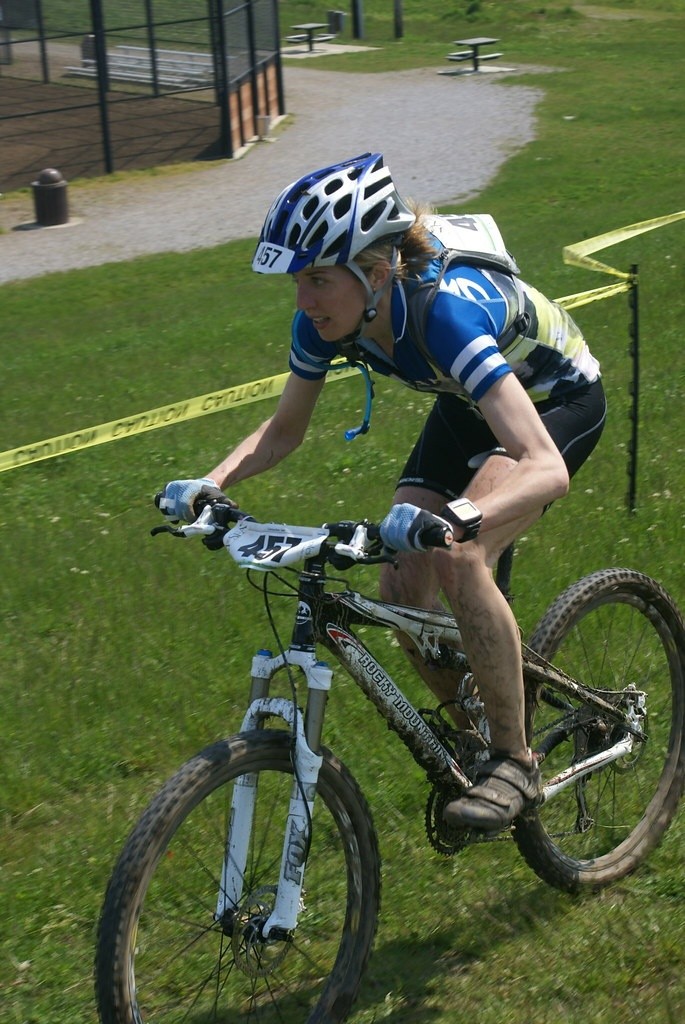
452;717;491;786
443;753;545;834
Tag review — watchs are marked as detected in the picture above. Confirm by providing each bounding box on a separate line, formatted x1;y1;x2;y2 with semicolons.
441;497;483;544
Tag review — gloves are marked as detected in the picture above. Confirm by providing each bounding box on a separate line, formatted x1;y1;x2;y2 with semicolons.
159;478;239;525
379;503;454;554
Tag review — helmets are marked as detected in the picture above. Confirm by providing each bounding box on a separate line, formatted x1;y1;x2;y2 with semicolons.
252;152;416;274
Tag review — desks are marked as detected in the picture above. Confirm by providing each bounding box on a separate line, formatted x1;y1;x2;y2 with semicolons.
453;36;501;71
290;23;331;50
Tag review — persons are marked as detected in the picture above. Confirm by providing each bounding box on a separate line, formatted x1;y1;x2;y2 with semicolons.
158;151;608;828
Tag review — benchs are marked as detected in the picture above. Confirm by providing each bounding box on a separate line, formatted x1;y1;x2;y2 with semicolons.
286;33;308;45
475;52;504;62
311;33;338;43
444;50;474;61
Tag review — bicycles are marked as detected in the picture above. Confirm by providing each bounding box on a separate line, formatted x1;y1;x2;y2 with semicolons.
93;485;685;1024
